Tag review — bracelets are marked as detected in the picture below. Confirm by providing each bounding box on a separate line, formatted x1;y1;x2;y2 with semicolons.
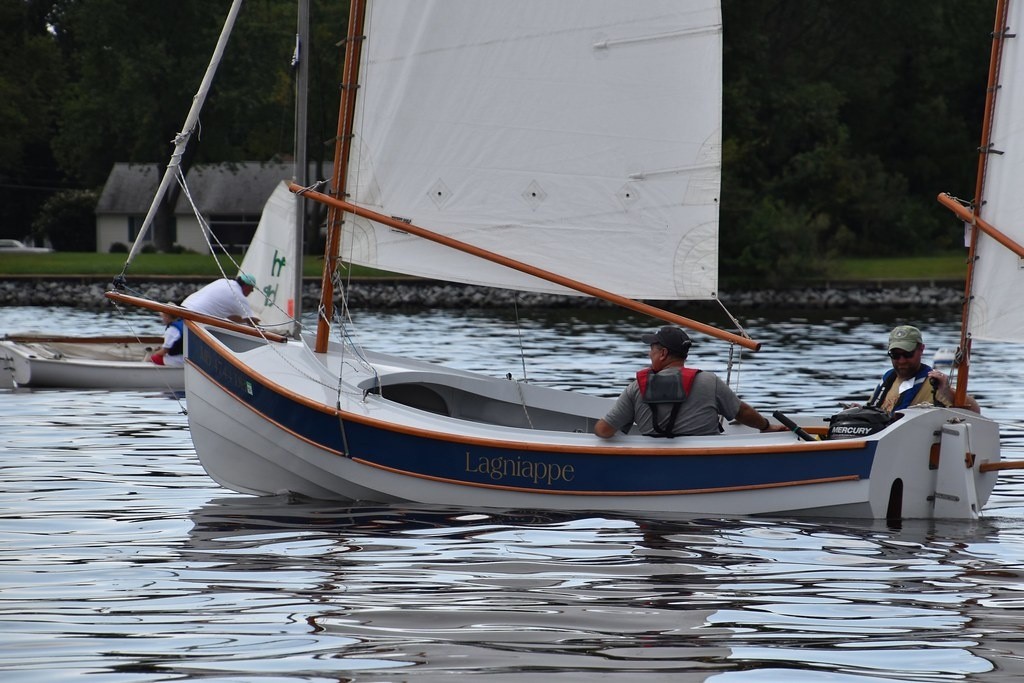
761;418;770;430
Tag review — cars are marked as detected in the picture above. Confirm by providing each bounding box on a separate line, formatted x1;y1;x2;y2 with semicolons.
0;239;52;250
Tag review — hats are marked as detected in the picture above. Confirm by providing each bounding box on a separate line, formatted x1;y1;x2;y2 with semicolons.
240;273;256;287
642;327;692;354
887;325;922;354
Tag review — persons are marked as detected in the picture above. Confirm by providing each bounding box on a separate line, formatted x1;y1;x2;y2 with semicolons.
851;324;981;416
142;311;184;367
182;272;261;328
594;326;788;438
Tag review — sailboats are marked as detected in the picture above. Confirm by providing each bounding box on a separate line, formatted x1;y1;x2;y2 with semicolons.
0;0;313;395
100;0;1024;523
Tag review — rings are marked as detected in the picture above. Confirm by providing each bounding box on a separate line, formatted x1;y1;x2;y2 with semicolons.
941;374;944;376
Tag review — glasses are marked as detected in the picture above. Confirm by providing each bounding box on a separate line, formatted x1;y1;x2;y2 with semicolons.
888;348;918;360
